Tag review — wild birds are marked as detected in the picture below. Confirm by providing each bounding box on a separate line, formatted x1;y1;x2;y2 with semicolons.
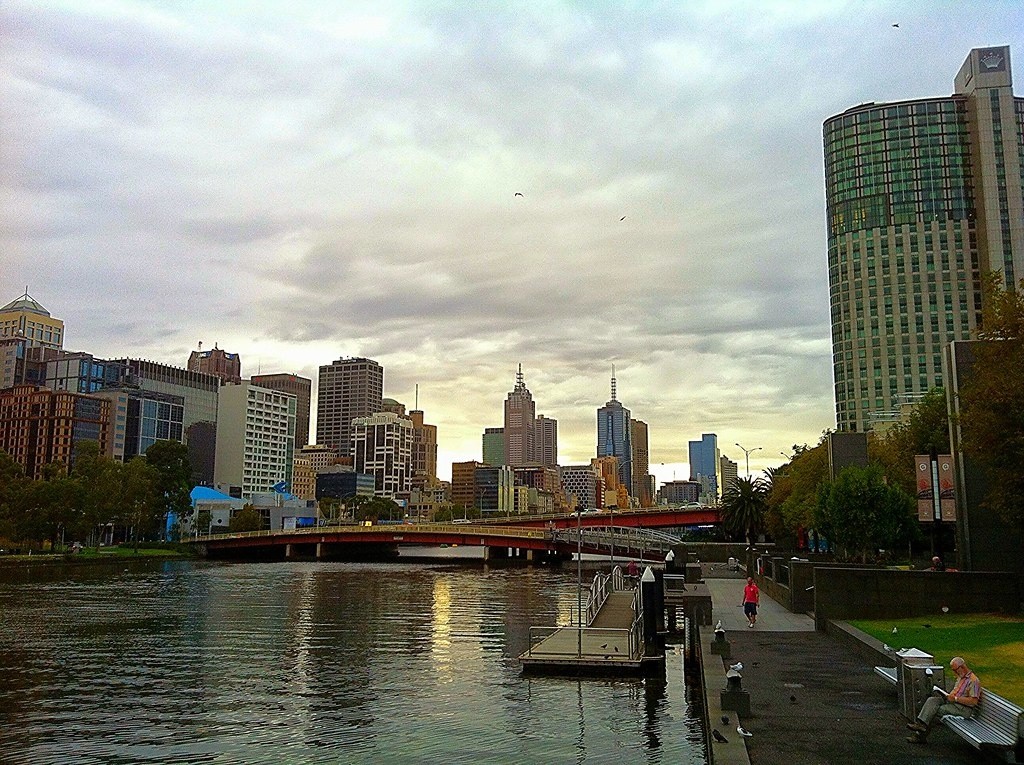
601;644;607;649
922;624;931;628
892;627;898;634
892;23;899;27
736;725;752;738
730;662;743;672
620;216;626;221
790;695;796;704
515;193;523;197
880;643;893;654
615;646;619;652
715;620;722;630
721;715;729;726
693;585;697;590
698;546;769;571
713;729;728;743
752;661;760;666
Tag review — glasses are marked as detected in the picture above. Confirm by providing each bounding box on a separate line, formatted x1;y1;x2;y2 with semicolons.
952;666;960;671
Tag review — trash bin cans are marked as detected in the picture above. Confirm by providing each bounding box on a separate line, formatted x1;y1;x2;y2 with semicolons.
895;647;945;724
728;557;735;569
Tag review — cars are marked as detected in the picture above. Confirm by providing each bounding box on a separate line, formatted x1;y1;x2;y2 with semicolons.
681;502;708;510
570;509;618;517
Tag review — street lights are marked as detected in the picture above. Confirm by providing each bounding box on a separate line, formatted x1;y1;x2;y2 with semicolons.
735;443;762;477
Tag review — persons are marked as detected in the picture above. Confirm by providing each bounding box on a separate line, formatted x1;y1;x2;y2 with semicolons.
627;560;638;590
932;557;945;571
741;577;760;628
906;657;981;743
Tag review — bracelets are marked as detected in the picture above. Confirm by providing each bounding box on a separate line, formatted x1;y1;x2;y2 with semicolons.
955;697;957;702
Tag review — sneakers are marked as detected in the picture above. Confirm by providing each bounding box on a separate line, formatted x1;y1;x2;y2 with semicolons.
748;622;754;628
907;723;926;732
906;734;927;744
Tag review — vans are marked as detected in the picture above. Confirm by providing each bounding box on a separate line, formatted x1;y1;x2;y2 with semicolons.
452;519;472;525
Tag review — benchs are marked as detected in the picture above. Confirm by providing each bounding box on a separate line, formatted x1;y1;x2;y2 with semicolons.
938;687;1024;765
874;666;897;697
